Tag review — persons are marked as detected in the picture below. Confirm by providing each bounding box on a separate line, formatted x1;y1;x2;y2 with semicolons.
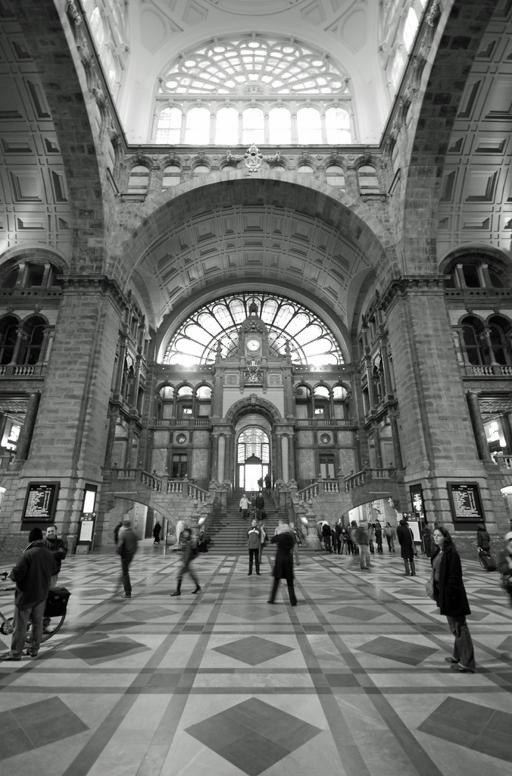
114;522;123;554
43;524;68;634
112;520;137;598
153;522;161;542
427;526;477;673
239;474;300;575
265;523;298;607
496;531;512;607
169;528;201;596
316;518;440;577
0;526;54;662
476;523;491;556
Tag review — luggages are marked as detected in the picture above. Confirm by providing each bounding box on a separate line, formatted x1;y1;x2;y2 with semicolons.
479;549;496;571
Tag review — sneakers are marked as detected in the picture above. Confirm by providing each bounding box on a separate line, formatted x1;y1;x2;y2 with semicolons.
0;648;38;661
446;656;475;673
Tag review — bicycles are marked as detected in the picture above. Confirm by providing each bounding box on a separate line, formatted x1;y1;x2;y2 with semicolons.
1;572;69;645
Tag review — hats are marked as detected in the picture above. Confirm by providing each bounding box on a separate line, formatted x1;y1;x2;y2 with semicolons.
28;528;44;543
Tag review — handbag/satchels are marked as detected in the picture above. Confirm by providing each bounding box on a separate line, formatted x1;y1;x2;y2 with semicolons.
425;578;435;598
411;542;417;554
43;587;71;617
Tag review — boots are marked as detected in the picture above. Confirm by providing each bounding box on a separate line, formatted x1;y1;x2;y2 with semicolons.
170;581;182;596
191;581;201;593
